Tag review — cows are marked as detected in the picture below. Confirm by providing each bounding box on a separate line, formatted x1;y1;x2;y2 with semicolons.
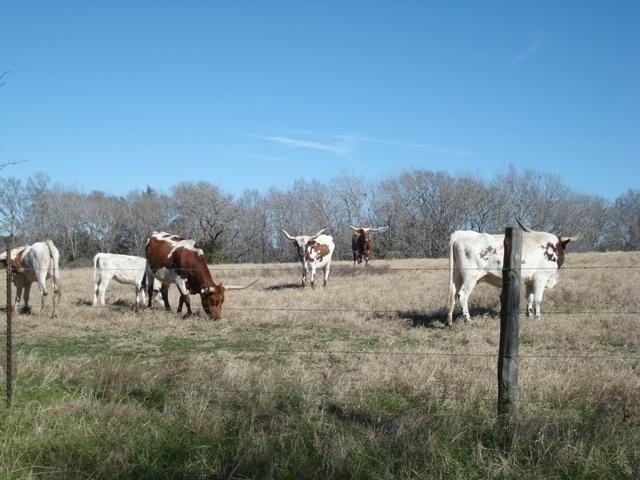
139;229;260;323
0;239;63;318
443;210;579;327
274;227;337;288
344;222;389;265
90;251;163;307
